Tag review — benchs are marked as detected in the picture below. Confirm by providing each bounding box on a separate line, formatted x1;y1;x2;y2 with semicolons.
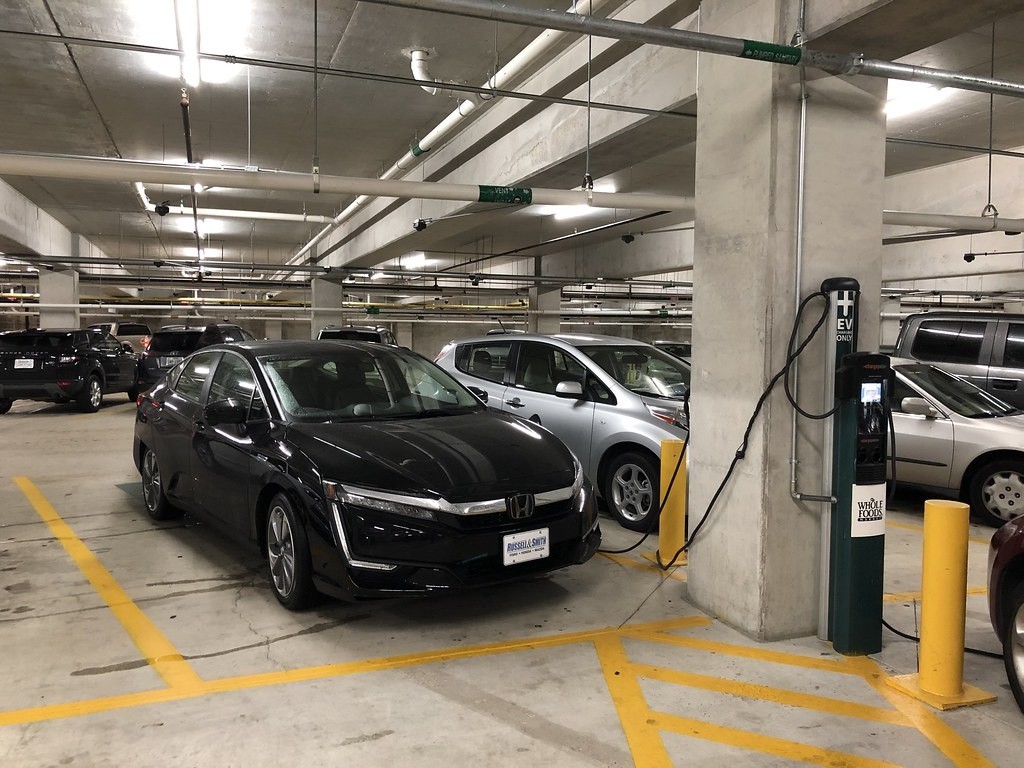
278;368;342;410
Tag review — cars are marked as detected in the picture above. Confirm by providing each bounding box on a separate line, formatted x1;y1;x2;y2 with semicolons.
131;338;604;611
879;354;1024;528
635;338;692;406
985;514;1024;714
410;330;691;533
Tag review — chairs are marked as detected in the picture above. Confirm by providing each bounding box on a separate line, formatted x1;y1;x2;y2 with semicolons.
217;370;264;408
472;350;503;382
517;347;555;393
337;370;373;403
591;352;625;396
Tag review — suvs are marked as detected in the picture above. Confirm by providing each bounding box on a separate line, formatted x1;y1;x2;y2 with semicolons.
876;312;1024;408
0;326;138;415
86;322;153;356
310;323;407;388
128;323;281;402
